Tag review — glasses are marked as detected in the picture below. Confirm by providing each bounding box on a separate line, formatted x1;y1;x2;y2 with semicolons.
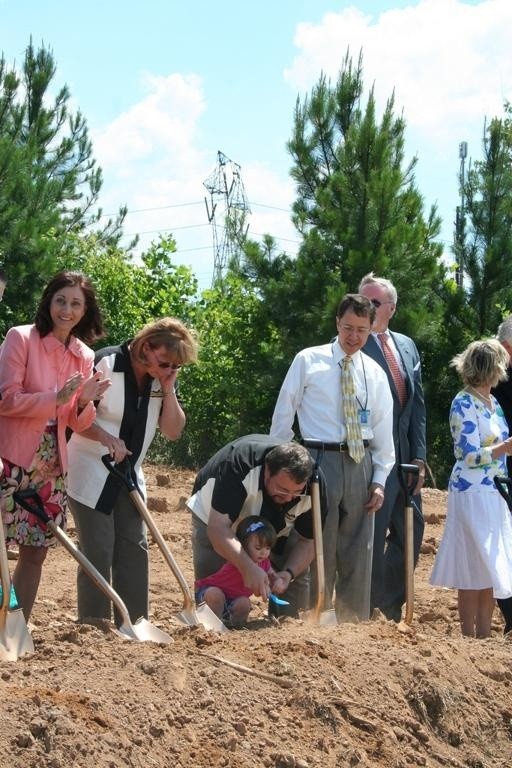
371;299;394;309
151;348;182;369
273;483;308;497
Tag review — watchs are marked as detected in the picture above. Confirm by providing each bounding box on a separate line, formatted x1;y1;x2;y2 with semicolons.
285;566;296;583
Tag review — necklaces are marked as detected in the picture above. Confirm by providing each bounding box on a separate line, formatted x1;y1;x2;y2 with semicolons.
470;385;494;403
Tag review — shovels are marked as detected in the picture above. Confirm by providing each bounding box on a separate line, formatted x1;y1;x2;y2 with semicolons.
102;453;230;635
0;511;34;662
299;439;337;626
13;489;174;644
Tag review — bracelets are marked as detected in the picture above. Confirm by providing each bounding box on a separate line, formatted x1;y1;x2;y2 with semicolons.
162;388;176;398
504;440;511;452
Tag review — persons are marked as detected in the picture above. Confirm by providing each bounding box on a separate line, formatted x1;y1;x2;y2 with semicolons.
186;433;328;618
491;318;512;638
62;317;196;633
427;338;512;639
328;270;428;622
1;272;113;623
270;292;395;626
194;515;284;629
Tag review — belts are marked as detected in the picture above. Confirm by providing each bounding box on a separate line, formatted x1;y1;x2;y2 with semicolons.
303;440;369;453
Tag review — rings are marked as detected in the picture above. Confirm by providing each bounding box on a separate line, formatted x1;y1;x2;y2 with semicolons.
69;386;73;392
378;501;383;507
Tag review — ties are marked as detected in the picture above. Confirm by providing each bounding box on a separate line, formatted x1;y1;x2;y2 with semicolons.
377;334;409;409
341;354;366;465
